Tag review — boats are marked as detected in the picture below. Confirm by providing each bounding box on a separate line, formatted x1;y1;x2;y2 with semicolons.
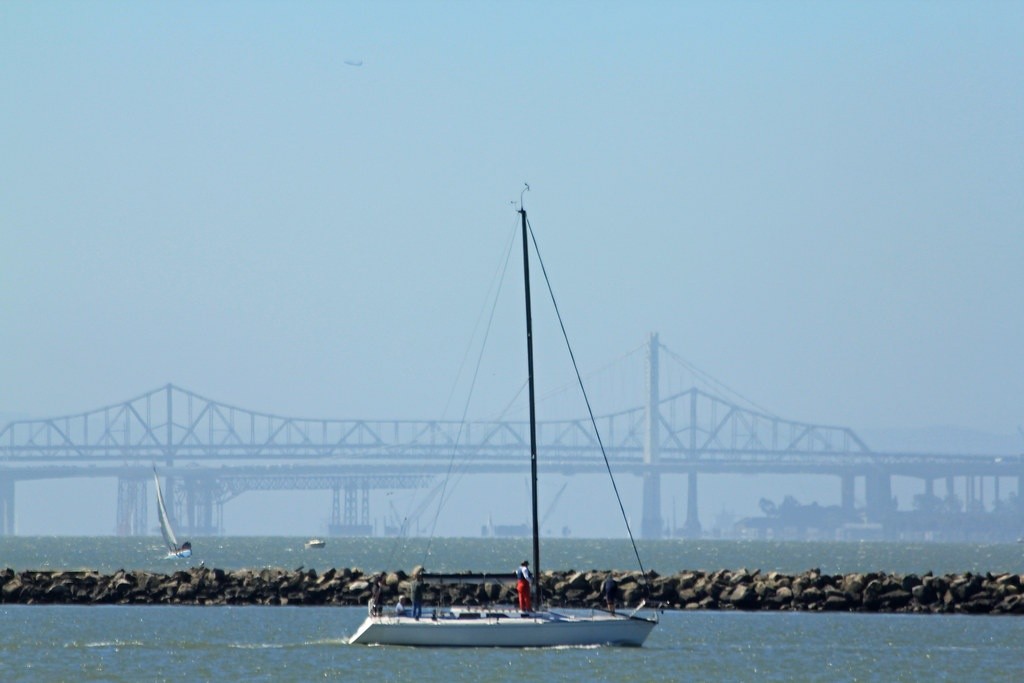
303;538;325;549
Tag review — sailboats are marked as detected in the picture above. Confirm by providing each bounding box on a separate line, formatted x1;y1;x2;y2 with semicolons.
349;182;660;651
151;467;192;562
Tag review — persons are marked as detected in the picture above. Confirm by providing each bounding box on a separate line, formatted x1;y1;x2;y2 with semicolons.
394;594;410;616
603;573;618;616
372;570;390;616
409;574;427;620
516;560;534;614
